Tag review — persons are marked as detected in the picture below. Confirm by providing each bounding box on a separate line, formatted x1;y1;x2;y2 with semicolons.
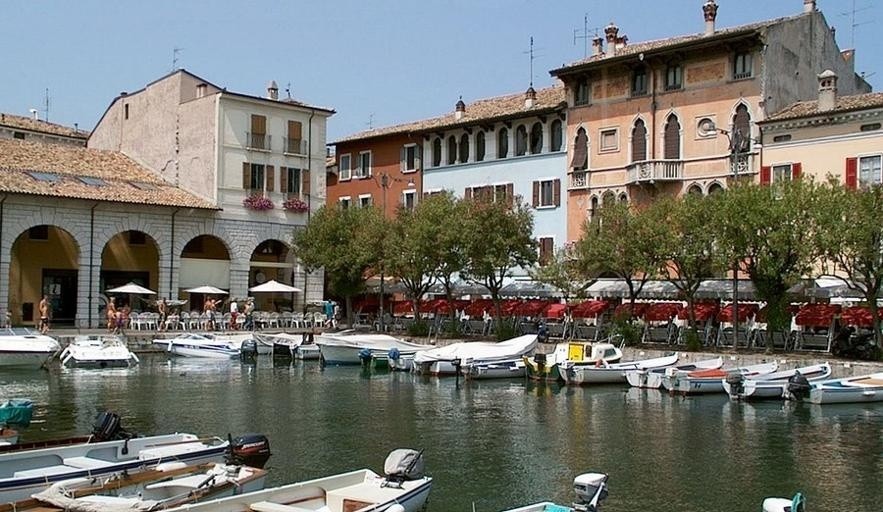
41;303;50;335
324;299;334;329
331;303;343;329
106;296;256;336
38;295;51;332
532;312;544;334
562;308;574;336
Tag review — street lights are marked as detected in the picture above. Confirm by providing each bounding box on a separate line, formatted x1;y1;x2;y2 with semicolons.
358;170;417;334
698;122;765;353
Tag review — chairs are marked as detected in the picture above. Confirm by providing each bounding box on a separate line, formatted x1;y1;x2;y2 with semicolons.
126;308;330;329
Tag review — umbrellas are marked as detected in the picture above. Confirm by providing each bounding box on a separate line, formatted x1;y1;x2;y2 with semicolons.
104;282;157;306
183;284;228;304
248;279;302;313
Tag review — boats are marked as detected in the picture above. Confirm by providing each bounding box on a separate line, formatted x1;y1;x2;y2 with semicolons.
60;335;141;370
756;491;808;512
502;470;614;512
0;326;66;374
